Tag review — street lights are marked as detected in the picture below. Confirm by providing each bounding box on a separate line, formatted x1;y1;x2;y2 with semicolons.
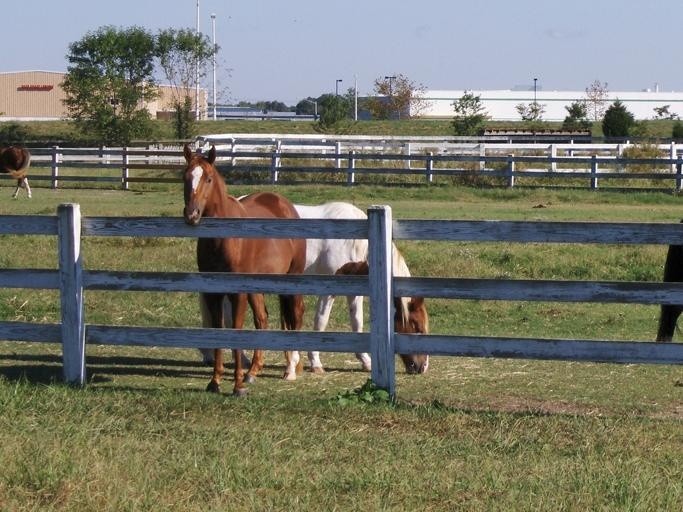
336;80;342;95
211;13;217;121
385;76;396;92
534;77;538;103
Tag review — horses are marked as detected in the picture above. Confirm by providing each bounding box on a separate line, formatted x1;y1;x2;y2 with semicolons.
0;145;32;199
196;200;431;376
179;142;307;398
